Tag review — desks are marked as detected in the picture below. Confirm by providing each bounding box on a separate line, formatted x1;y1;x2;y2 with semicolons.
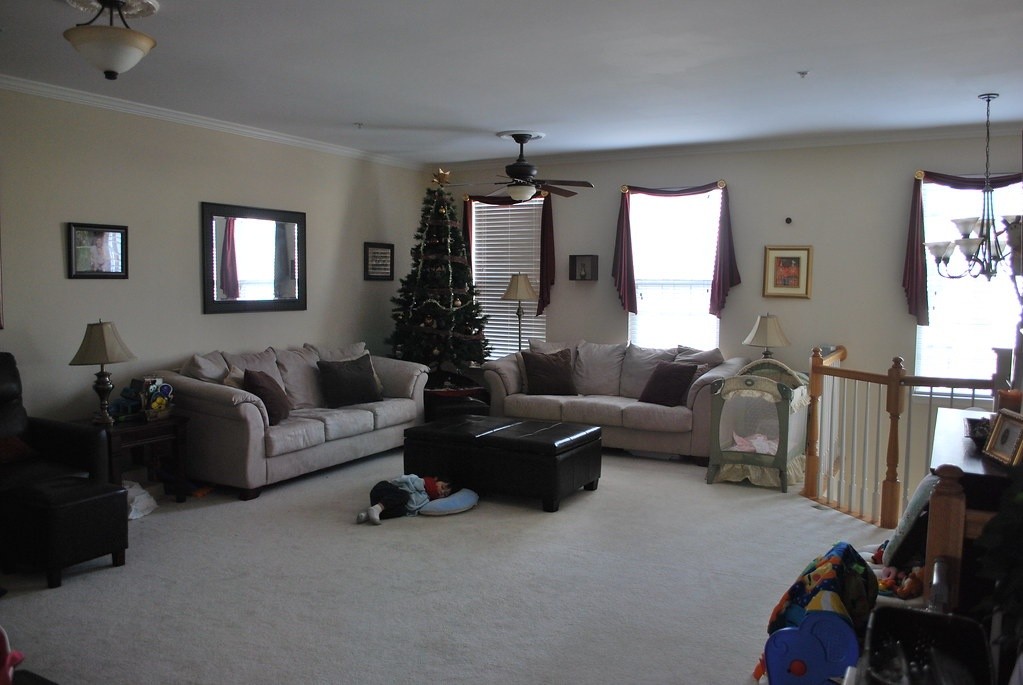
929;407;1017;515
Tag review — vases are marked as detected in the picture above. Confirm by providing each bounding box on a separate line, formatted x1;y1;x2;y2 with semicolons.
580;263;587;279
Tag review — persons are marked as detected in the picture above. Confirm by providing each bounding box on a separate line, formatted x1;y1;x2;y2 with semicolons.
356;474;455;525
90;237;105;272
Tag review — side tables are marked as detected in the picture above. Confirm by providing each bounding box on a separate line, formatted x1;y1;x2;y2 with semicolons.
68;411;190;496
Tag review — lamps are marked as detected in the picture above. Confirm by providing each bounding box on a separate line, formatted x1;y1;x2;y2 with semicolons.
507;184;537;202
68;319;137;424
498;271;540;351
740;312;791;360
63;0;160;81
922;93;1008;283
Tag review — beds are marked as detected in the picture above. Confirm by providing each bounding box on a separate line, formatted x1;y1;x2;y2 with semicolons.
706;358;810;493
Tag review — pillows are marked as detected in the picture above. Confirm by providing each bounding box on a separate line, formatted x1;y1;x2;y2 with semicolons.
315;354;384;409
327;349;384;393
528;338;586;372
268;343;323;410
304;342;367;361
521;348;578;396
244;369;289;426
884;472;939;567
572;338;628;396
638;360;698;407
515;349;563;394
684;363;708;402
619;341;680;399
674;346;725;372
180;349;230;384
221;347;288;397
223;366;246;390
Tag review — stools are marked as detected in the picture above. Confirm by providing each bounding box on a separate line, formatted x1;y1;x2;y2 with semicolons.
0;476;128;589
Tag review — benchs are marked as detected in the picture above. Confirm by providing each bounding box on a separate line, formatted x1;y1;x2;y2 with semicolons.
403;414;603;513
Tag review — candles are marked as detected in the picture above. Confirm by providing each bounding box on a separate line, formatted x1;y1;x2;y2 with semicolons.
998;379;1021;414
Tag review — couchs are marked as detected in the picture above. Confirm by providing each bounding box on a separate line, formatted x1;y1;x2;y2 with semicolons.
146;336;751;501
0;351;109;578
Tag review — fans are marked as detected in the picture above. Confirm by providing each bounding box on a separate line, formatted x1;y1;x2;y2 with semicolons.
485;130;595;198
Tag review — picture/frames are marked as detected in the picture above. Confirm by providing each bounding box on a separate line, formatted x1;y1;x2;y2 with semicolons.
982;406;1023;468
364;241;395;282
68;221;130;281
763;245;813;300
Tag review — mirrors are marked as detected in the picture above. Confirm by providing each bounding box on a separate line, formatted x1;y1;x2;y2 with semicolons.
200;202;307;314
